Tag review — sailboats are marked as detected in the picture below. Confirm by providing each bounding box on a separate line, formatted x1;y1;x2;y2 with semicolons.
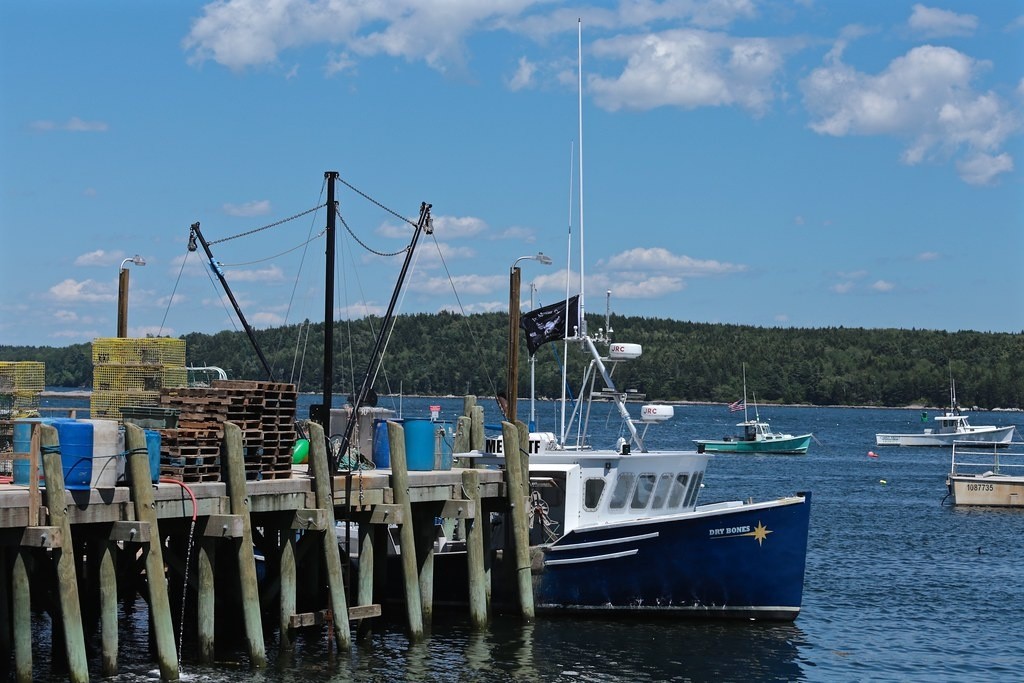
75;20;815;627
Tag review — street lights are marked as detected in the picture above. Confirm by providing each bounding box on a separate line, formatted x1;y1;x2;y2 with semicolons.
117;253;148;339
508;251;554;428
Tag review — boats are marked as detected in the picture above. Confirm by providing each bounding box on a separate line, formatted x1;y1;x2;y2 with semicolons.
875;358;1016;447
946;439;1024;509
691;362;815;454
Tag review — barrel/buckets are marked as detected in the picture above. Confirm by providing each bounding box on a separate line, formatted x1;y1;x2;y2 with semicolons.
404;417;436;470
435;420;453;472
371;418;403;469
12;418;161;490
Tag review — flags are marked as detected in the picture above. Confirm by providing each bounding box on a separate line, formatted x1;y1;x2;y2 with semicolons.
519;293;581;357
729;397;745;411
